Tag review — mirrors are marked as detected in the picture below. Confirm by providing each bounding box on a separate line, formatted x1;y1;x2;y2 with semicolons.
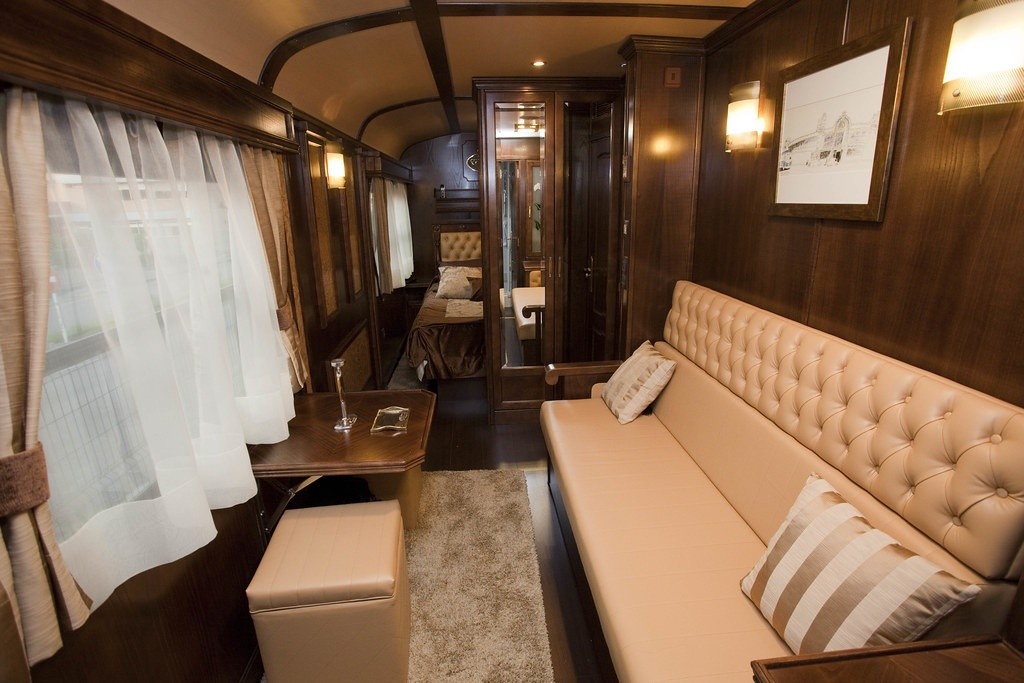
494;101;546;369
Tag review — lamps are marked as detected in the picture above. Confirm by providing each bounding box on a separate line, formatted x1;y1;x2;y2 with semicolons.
936;1;1023;115
325;140;346;190
726;79;761;151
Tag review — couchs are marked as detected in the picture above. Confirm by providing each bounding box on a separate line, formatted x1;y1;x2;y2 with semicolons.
539;276;1024;683
246;500;408;683
508;269;545;366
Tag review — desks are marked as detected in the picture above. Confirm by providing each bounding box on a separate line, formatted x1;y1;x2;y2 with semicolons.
238;390;437;553
521;260;545;286
748;635;1024;683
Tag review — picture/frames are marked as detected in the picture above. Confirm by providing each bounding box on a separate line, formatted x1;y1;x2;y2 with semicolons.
766;17;913;224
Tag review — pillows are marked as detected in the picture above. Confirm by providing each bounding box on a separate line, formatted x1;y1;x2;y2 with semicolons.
600;339;677;426
435;258;483;302
739;472;980;653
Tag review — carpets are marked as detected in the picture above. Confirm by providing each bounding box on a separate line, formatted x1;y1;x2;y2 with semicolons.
262;469;556;683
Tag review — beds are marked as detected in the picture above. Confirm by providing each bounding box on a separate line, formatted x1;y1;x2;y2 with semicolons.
405;223;487;391
